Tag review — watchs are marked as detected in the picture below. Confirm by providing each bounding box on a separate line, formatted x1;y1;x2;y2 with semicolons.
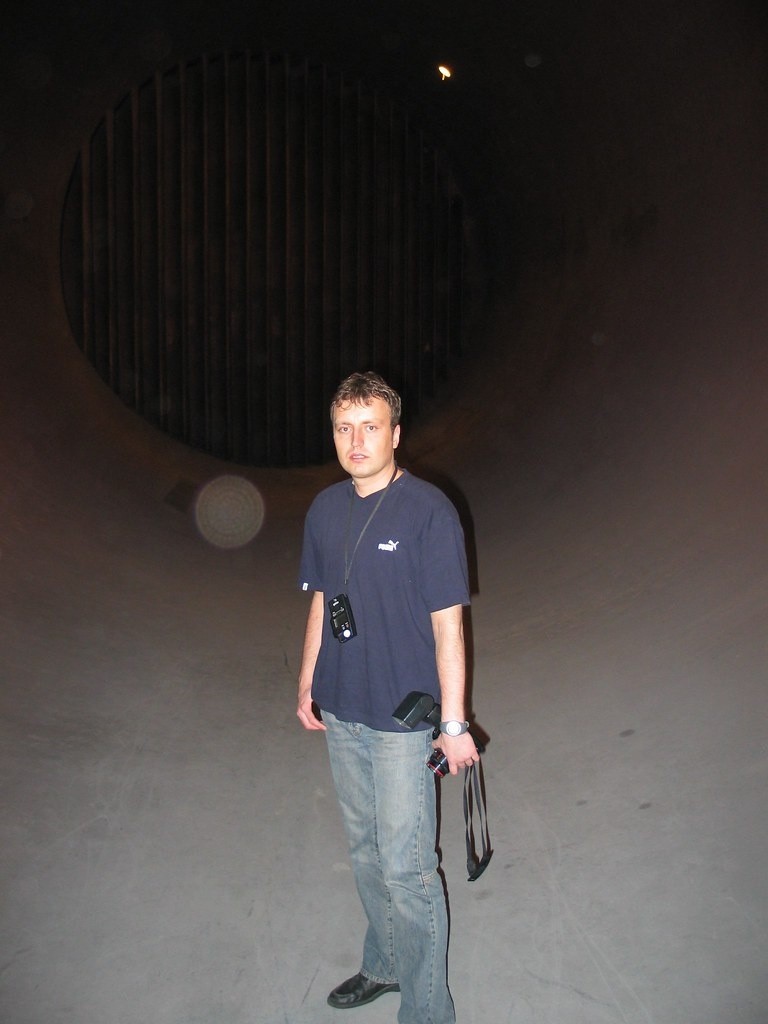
439;720;469;736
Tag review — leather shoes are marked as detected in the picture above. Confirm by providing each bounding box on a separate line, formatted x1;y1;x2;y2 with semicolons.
327;972;401;1009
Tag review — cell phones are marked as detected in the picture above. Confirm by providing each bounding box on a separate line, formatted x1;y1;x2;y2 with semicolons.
327;594;357;644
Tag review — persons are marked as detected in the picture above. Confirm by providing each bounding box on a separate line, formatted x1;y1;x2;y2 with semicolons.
298;370;479;1024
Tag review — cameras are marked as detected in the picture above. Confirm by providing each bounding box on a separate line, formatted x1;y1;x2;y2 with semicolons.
391;691;485;778
